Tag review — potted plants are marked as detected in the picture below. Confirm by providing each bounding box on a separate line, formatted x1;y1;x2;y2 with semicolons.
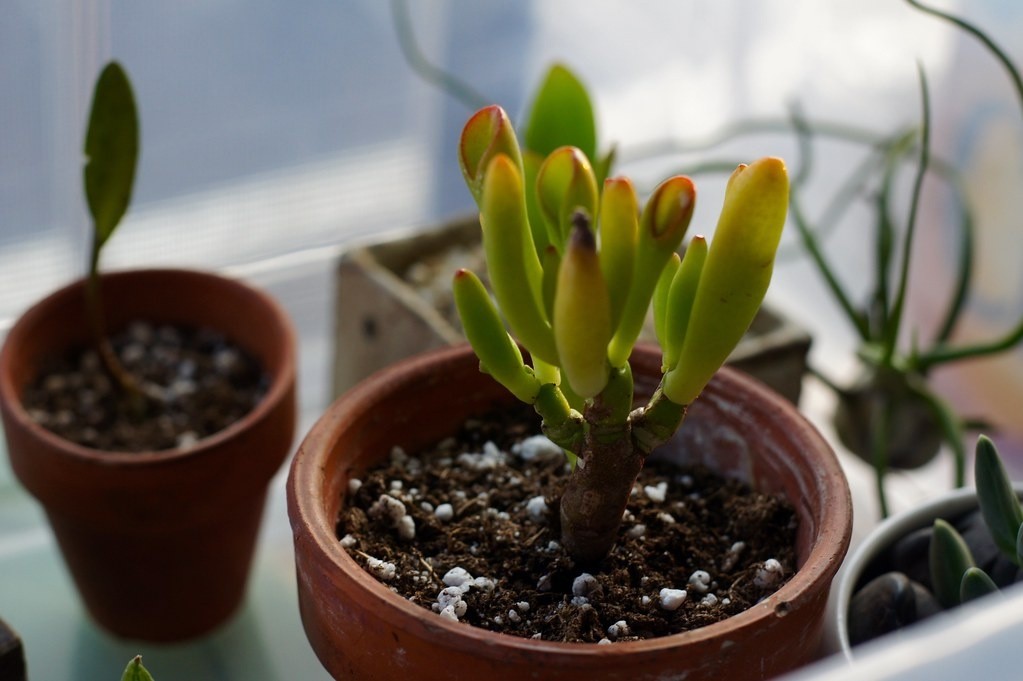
285;56;854;681
0;58;302;649
811;431;1023;662
329;1;814;412
